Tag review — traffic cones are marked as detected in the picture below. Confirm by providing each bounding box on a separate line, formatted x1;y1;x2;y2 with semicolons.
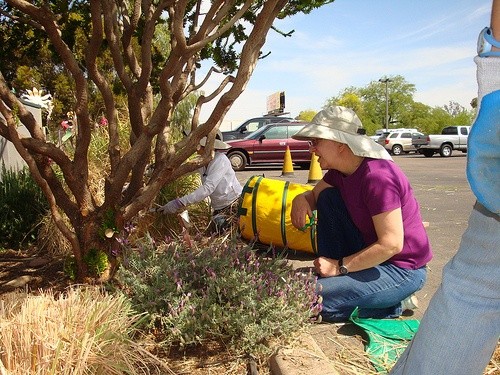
306;152;323;185
278;145;297;178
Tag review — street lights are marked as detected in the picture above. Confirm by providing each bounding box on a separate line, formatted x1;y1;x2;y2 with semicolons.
378;78;395;133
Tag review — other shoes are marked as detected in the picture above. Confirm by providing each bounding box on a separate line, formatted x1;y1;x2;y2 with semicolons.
405;294;418;310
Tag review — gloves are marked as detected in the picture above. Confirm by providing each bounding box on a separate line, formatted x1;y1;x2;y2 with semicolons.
157;199;183;216
467;27;500;217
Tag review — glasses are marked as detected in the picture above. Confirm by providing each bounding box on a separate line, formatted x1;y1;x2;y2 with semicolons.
307;138;324;146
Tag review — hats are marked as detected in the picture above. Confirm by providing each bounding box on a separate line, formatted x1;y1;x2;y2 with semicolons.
182;124;232;149
292;106;395;162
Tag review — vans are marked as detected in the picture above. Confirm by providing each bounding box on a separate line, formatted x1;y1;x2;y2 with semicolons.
367;128;425;155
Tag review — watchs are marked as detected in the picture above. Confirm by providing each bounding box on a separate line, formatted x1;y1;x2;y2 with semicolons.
477;26;500;57
338;257;348;274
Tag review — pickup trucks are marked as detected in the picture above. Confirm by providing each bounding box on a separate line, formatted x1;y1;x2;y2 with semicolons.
411;125;472;157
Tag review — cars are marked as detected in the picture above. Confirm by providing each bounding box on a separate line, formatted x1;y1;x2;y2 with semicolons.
220;116;295;143
214;122;317;171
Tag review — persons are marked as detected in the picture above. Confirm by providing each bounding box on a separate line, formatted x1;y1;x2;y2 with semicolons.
157;123;243;238
386;0;500;375
291;105;433;324
